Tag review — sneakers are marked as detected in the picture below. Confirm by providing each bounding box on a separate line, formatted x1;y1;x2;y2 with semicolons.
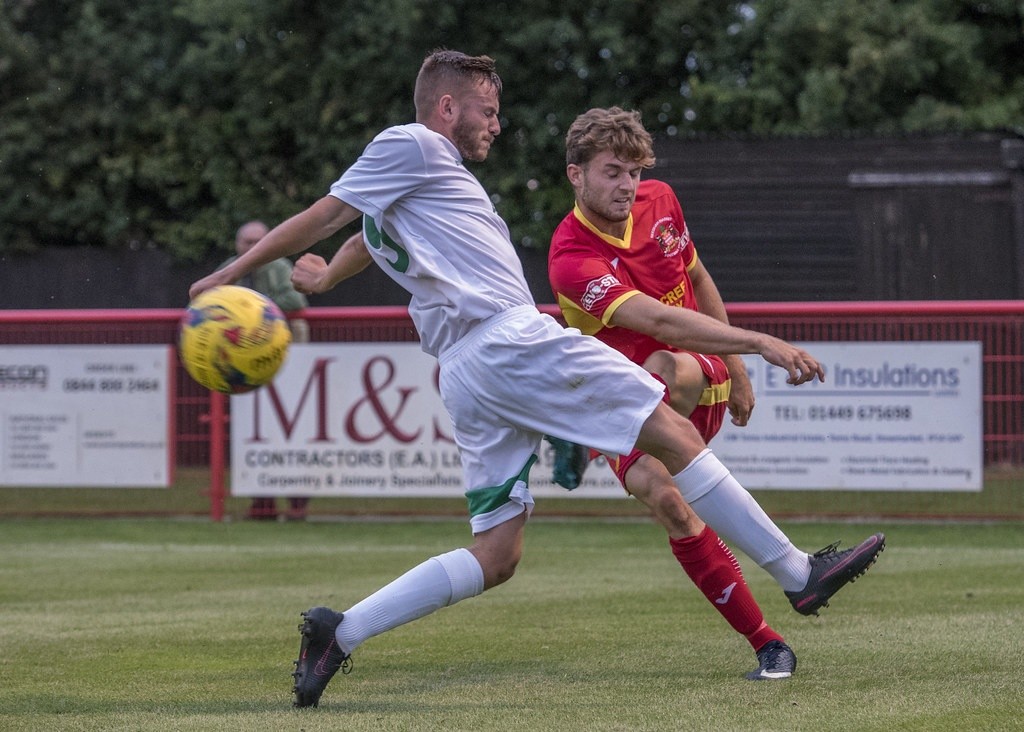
747;641;796;679
290;607;354;710
544;434;588;490
785;531;886;618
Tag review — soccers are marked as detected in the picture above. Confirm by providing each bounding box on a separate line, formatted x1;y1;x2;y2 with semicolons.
174;285;291;396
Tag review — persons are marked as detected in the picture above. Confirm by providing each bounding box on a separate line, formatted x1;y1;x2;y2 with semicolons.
189;49;885;708
548;107;825;679
214;221;311;523
